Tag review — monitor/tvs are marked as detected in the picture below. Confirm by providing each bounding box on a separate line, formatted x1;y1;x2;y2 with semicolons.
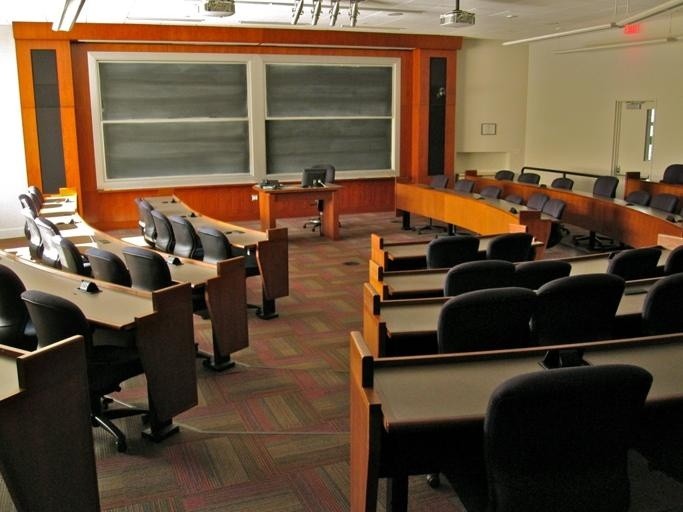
302;169;326;187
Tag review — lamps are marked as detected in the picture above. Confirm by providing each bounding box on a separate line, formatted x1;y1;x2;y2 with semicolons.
286;1;359;29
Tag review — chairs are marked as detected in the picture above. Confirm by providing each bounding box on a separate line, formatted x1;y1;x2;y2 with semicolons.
303;165;343;234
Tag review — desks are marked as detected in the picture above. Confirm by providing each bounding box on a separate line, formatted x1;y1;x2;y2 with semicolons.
0;334;103;511
252;180;343;243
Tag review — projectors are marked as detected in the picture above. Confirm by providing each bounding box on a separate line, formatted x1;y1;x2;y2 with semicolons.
196;0;235;18
440;13;475;27
